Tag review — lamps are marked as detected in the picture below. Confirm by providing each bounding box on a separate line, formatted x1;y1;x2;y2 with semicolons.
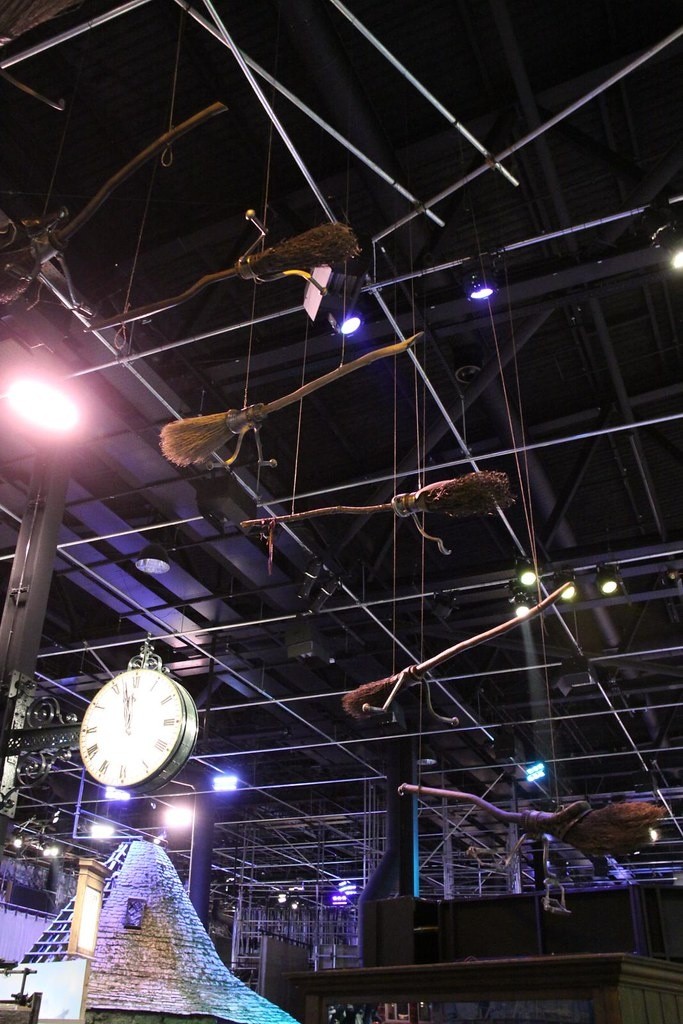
301;262;365;335
135;538;170;575
504;581;537;616
553;565;577;600
514;554;540;586
639;201;683;268
461;260;499;301
596;562;622;597
432;588;460;619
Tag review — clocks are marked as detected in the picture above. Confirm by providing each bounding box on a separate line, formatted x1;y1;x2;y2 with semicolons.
79;632;199;795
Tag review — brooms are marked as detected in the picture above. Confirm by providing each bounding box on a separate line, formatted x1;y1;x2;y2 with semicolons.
157;331;424;468
0;1;84;49
0;100;229;305
398;784;674;880
340;581;575;724
82;221;363;333
239;471;517;528
